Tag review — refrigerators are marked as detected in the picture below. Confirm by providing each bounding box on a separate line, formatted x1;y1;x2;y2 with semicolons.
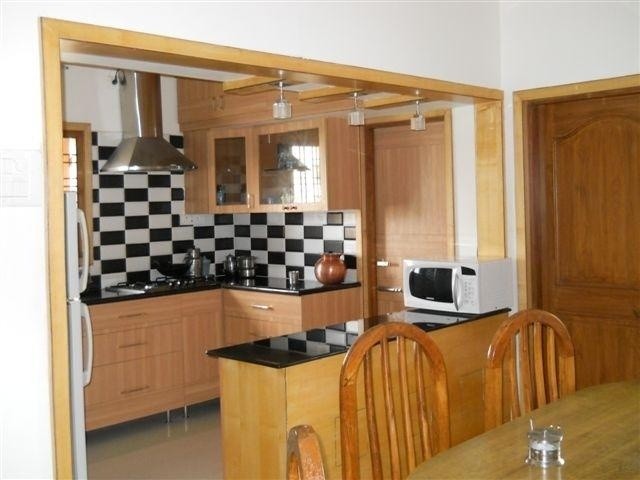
65;188;95;479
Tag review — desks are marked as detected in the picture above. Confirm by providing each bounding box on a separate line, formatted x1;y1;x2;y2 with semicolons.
407;379;640;480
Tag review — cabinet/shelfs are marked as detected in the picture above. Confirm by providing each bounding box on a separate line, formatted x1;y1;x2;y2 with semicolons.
183;116;360;214
83;275;363;432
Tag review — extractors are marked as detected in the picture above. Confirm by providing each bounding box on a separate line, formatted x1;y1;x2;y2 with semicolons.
99;69;199;177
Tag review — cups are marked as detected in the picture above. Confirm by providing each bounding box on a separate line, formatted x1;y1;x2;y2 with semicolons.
524;417;567;468
288;270;300;287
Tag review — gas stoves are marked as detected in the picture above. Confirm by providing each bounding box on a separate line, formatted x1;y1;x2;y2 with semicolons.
100;275;218;294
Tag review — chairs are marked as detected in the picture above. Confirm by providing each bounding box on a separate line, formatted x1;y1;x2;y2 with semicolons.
338;321;451;480
286;423;326;480
484;309;576;433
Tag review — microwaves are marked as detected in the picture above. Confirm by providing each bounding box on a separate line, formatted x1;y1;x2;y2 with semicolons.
402;258;519;320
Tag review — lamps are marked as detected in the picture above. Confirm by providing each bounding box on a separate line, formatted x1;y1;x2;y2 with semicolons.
348;92;365;126
272;81;292;119
411;100;425;131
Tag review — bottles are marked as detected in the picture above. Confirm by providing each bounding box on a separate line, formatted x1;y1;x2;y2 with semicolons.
182;245;258;280
